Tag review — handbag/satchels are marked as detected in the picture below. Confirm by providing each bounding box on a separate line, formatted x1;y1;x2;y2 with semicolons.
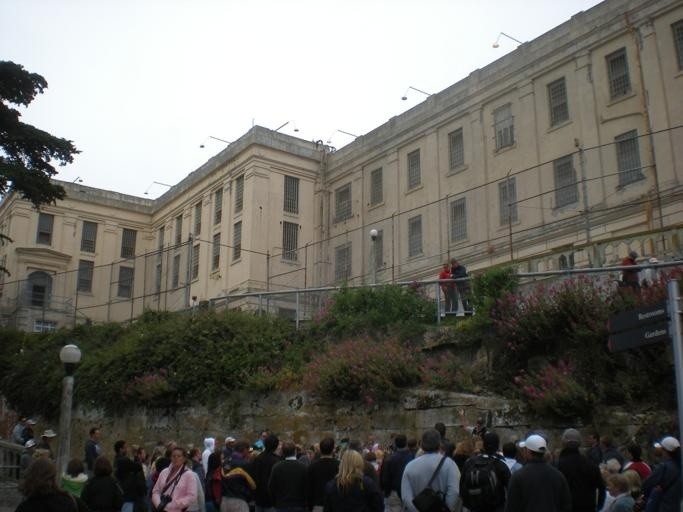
411;486;450;512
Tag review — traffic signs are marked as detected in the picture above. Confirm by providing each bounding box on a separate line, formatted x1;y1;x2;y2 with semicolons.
606;321;668;354
606;304;668;335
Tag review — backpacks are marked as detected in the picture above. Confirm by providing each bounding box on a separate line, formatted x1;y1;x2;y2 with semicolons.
460;455;499;510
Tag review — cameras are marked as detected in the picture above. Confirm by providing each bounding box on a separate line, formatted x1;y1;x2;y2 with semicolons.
156;494;172;512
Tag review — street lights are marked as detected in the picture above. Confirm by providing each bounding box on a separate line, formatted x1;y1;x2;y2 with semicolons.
369;230;378;292
55;344;83;491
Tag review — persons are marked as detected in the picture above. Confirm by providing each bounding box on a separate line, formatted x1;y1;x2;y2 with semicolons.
622;251;640;293
450;258;473;317
12;415;683;512
440;264;458;316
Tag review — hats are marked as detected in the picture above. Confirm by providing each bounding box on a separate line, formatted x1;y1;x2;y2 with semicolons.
518;426;582;454
656;437;681;452
23;418;56;449
